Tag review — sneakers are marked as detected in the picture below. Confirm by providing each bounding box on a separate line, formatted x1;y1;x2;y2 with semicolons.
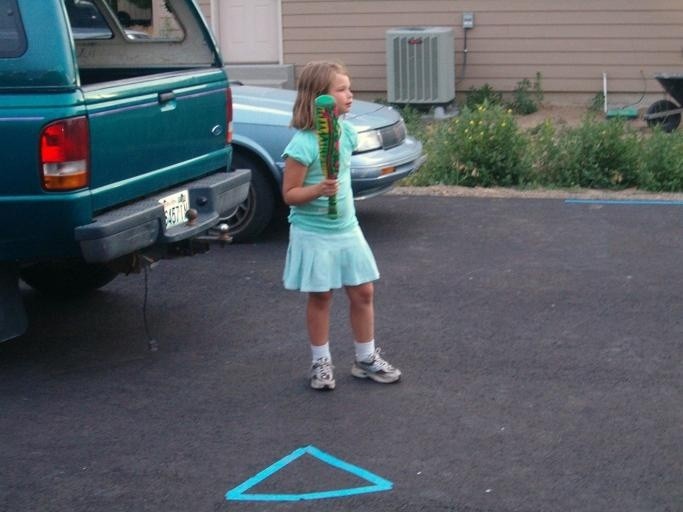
352;347;401;383
311;357;336;390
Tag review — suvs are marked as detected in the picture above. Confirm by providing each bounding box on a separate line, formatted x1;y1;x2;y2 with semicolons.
1;1;252;294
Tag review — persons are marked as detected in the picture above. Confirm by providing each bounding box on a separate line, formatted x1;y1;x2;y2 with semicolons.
281;60;403;391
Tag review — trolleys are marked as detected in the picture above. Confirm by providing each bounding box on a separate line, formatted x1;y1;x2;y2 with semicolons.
643;74;682;130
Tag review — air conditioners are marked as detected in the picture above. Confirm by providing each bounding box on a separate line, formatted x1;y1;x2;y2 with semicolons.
385;26;456;104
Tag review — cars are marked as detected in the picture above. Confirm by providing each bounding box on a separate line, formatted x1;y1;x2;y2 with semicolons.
209;86;423;243
